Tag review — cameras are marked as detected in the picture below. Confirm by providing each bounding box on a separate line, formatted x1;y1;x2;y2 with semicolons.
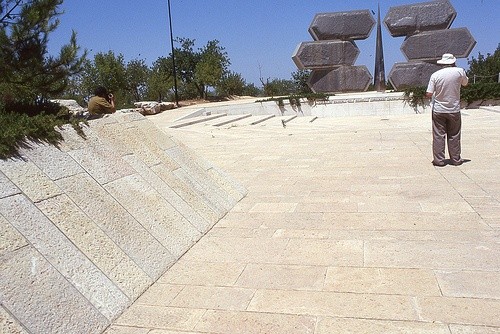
106;94;112;100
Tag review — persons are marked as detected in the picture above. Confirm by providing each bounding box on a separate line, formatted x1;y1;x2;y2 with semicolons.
87;87;117;118
426;53;468;166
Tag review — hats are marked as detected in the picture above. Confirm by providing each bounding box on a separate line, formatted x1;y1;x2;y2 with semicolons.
436;54;457;65
